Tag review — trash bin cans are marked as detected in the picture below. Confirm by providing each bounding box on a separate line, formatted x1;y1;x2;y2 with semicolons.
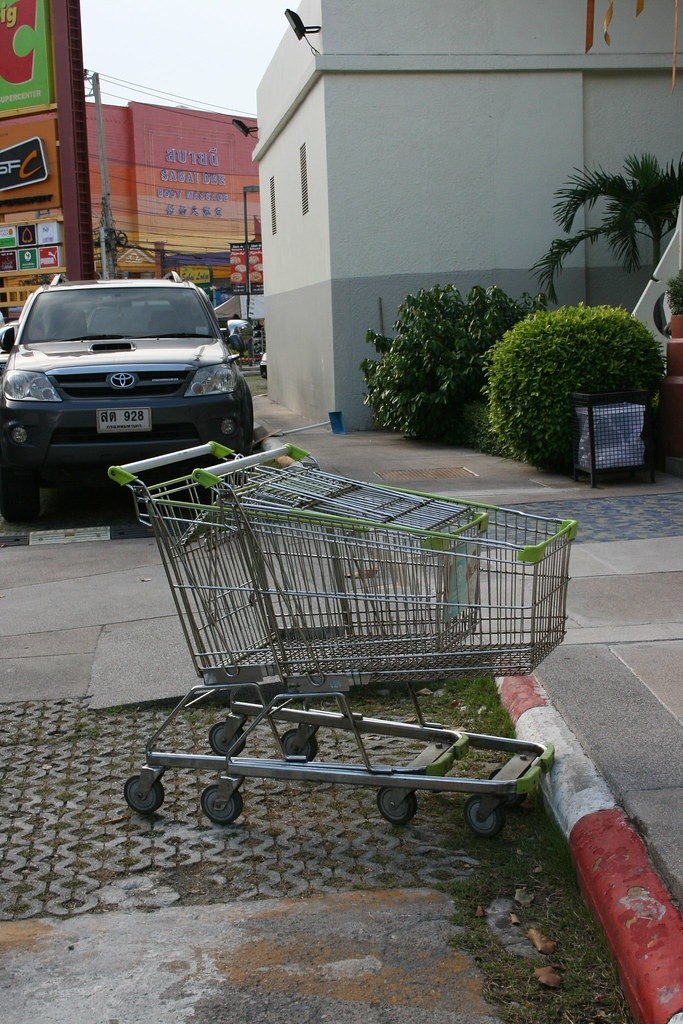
565;387;657;489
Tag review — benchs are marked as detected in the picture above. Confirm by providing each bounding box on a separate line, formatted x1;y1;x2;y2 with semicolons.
86;307;179;335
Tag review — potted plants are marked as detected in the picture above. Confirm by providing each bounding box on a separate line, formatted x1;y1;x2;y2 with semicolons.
665;268;683;339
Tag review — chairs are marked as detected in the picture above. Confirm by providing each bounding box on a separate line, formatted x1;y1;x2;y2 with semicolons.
44;305;88;340
147;308;198;336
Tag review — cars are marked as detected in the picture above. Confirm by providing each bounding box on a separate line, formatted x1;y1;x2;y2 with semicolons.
0;319;21;374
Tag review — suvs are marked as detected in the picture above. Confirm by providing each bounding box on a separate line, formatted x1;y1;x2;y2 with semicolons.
0;270;258;523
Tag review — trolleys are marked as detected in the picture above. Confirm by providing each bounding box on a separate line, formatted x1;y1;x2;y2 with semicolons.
189;443;578;839
107;437;470;824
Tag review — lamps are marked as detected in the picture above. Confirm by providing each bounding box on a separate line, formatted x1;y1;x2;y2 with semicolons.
285;9;324;56
230;118;260;141
